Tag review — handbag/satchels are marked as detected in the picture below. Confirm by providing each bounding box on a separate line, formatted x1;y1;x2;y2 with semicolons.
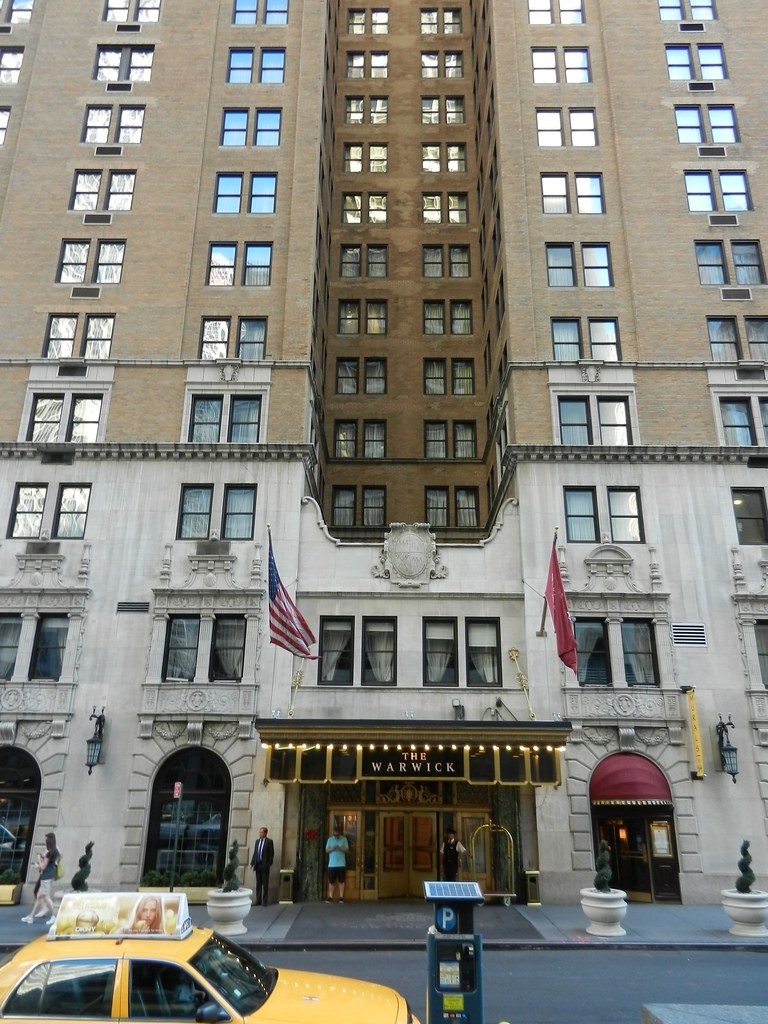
54;860;64;881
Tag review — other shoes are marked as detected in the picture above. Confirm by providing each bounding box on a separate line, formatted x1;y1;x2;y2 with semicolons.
338;898;344;904
325;898;333;904
35;908;49;918
253;902;262;906
262;902;267;906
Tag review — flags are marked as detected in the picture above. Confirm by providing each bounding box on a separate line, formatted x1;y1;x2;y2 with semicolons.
544;542;578;675
267;530;321;661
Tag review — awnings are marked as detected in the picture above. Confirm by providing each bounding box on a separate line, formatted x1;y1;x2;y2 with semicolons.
590;752;674;806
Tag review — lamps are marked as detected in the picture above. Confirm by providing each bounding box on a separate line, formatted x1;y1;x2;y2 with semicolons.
451;696;466;720
81;704;109;776
714;710;742;786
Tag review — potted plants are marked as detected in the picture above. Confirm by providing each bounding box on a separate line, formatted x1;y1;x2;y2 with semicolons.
52;841;102;918
720;838;768;938
137;871;222;905
0;868;23;906
205;839;254;936
579;839;627;936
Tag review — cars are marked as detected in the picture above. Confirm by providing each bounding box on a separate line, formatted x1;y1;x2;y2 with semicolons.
1;890;423;1024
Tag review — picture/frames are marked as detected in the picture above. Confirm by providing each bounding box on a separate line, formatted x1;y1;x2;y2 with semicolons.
649;823;673;858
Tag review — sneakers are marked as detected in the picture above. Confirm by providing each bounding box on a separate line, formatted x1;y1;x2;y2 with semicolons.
46;916;55;925
22;916;33;924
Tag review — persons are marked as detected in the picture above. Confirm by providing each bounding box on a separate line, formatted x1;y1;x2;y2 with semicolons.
250;827;274;906
20;832;60;925
326;827;349;904
114;895;162;934
440;828;472;882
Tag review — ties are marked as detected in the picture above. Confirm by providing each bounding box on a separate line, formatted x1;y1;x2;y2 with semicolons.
259;839;263;859
450;839;451;842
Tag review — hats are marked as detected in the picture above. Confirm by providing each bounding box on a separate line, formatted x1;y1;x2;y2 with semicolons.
445;829;457;834
